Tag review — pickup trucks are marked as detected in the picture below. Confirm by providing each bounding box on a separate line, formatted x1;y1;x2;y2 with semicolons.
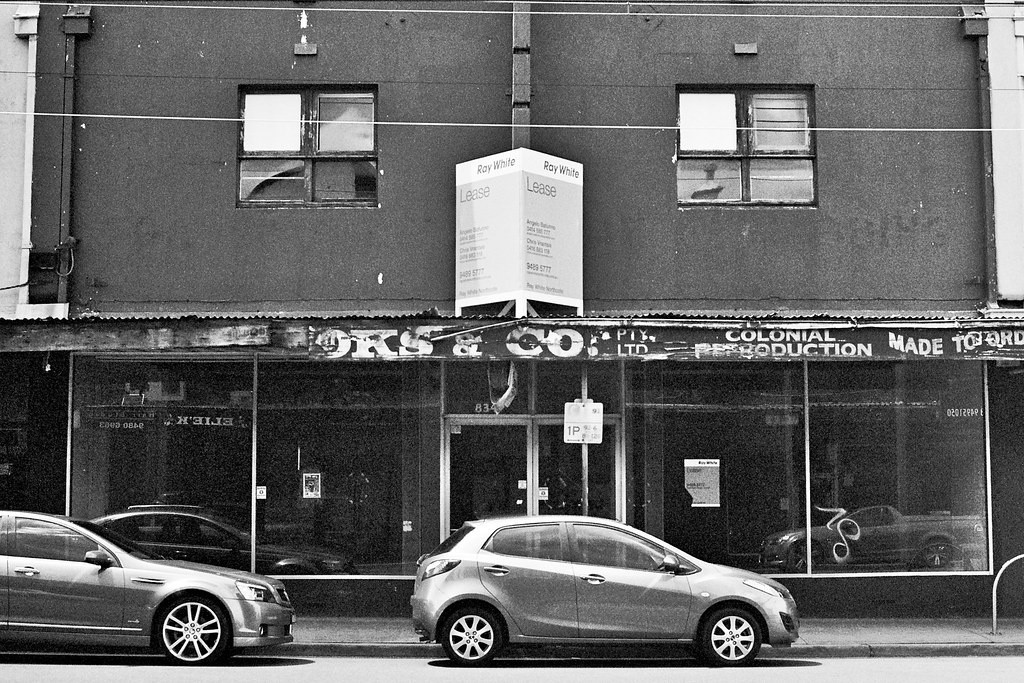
750;504;984;569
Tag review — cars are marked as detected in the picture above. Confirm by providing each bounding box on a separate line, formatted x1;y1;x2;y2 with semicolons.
90;503;359;579
412;511;803;670
0;508;295;665
